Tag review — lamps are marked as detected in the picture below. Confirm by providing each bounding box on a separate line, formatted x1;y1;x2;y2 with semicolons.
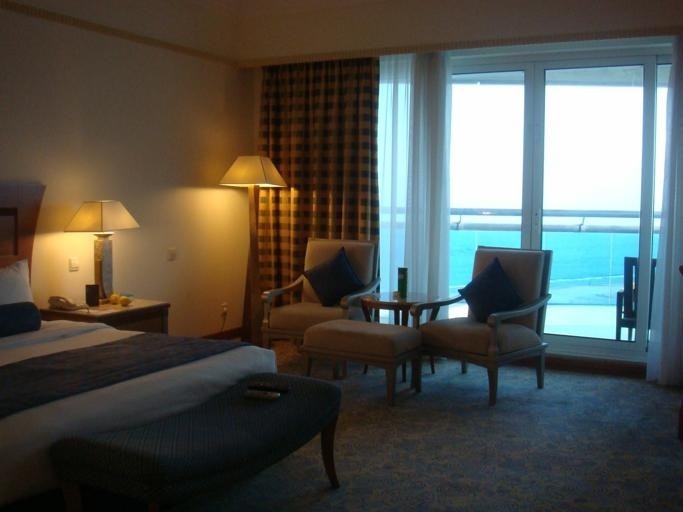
65;199;141;300
218;154;288;350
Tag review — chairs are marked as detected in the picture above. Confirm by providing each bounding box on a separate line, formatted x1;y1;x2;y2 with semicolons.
417;247;552;402
614;257;658;342
260;238;379;379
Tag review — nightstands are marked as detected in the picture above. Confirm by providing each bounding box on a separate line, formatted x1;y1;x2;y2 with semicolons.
43;296;170;338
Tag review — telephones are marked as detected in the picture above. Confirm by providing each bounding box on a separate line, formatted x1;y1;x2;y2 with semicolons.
47;296;83;311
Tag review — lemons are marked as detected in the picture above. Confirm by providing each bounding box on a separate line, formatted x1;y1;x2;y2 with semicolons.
110;295;118;305
119;296;132;306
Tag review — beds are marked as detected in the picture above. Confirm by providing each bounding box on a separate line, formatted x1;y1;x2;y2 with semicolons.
0;184;280;511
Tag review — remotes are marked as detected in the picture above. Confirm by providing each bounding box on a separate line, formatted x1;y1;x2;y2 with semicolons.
245;389;280;401
248;380;288;391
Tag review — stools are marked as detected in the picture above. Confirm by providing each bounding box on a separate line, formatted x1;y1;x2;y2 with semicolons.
299;320;422;399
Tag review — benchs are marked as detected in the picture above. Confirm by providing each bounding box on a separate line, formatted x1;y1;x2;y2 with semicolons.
77;368;344;511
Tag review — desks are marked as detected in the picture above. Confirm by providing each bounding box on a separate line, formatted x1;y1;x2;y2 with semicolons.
362;291;464;386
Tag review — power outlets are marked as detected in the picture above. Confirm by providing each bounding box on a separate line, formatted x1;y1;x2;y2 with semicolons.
220;304;230;318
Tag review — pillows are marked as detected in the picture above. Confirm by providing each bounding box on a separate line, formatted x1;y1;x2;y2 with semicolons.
0;257;39;338
458;258;519;321
301;247;366;308
